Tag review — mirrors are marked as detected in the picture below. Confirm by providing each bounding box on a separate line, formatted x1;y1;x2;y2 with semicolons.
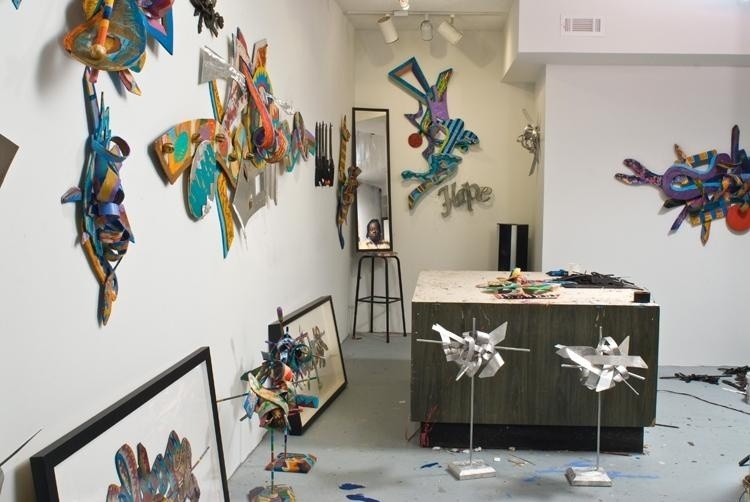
350;104;395;253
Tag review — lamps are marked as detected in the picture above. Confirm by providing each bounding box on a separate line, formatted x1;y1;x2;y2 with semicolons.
376;8;465;46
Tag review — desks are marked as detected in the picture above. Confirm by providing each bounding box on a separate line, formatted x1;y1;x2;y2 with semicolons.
407;266;663;457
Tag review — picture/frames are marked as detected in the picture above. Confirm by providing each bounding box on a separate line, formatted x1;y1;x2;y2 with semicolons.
264;293;348;437
27;342;232;502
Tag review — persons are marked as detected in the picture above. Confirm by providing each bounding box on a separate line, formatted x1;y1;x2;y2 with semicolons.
359;219;390;250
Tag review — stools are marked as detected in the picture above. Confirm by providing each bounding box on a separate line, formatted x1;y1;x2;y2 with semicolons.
347;250;408;344
